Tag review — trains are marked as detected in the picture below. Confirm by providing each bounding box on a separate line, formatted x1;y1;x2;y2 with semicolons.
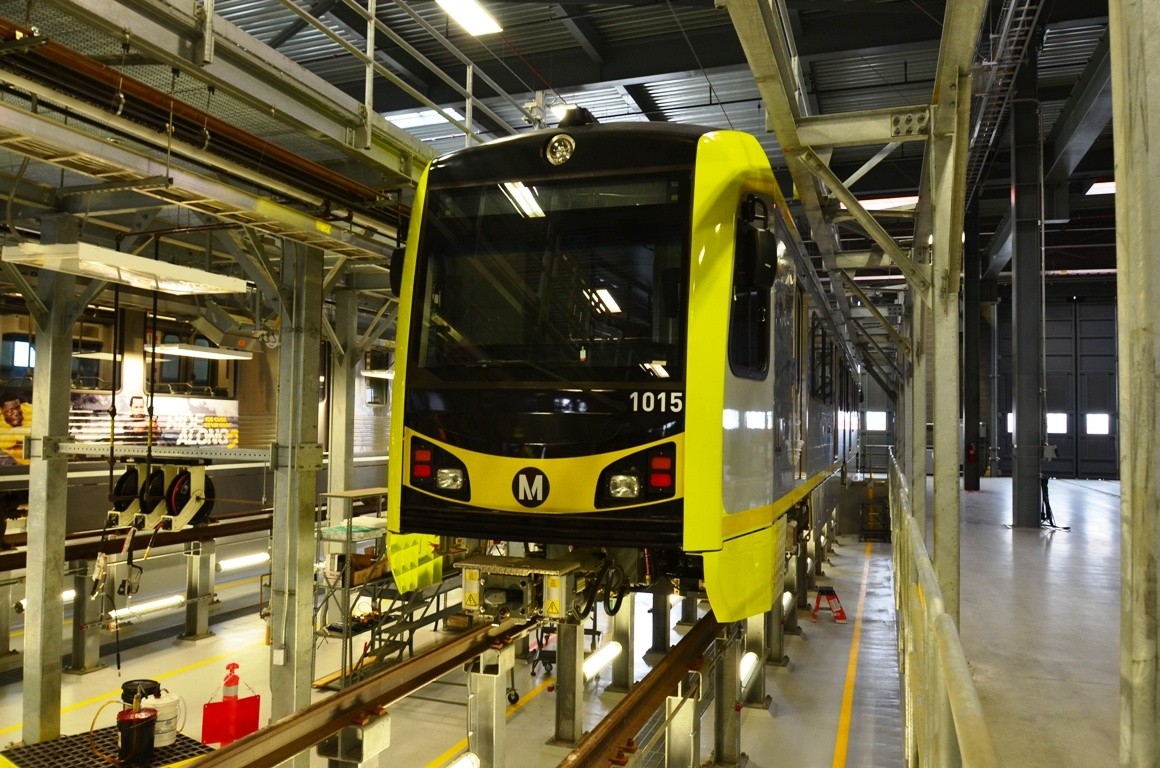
386;106;859;626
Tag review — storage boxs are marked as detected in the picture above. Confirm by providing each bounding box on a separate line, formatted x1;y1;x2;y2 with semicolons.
351;553;383;587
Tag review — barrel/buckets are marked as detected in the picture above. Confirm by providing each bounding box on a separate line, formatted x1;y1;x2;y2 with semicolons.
116;679;181;763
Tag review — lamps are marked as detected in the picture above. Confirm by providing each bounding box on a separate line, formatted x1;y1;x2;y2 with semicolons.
144;343;253;360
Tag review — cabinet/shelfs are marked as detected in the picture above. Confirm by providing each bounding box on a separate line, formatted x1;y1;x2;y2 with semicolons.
311;488;510;692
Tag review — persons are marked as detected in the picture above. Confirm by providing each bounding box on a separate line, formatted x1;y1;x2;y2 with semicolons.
0;394;33;465
123;396;162;444
